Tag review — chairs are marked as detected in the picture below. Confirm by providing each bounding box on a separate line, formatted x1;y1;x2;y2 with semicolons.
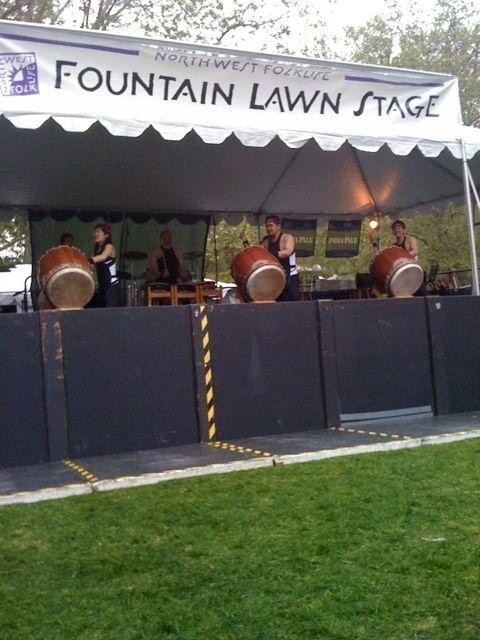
148;284;224;306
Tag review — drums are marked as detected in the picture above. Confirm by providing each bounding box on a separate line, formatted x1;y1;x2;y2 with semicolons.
231;245;286;303
369;246;424;295
36;245;96;308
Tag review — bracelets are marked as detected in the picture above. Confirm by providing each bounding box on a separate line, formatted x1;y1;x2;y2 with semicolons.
88;257;94;264
372;242;377;247
243;240;250;247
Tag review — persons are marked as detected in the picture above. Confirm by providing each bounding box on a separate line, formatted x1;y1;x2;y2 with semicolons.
370;219;419;263
149;230;187;283
87;223;121;307
239;215;301;301
60;233;74;246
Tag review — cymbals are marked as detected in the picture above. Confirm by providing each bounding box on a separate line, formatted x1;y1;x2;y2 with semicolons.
123;252;148;261
183;252;203;260
116;270;137;281
